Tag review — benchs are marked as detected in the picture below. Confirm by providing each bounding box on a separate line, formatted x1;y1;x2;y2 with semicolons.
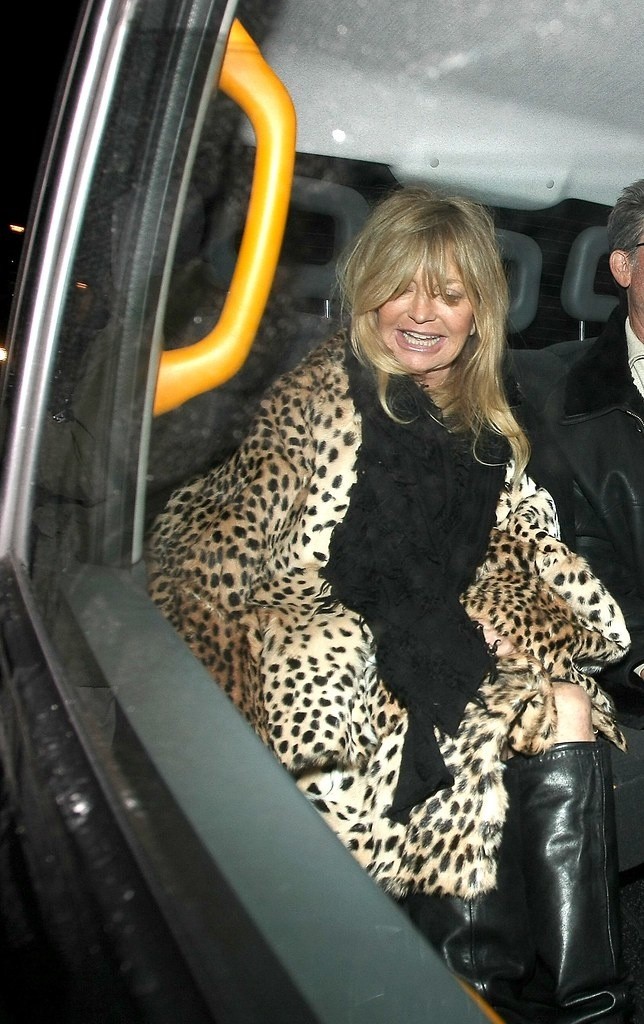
174;167;643;879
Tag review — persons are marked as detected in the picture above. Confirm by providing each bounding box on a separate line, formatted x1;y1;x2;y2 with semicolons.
505;179;644;690
139;184;632;1024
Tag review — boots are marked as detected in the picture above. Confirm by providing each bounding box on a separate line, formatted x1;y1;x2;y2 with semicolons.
443;757;531;1024
516;741;633;1024
